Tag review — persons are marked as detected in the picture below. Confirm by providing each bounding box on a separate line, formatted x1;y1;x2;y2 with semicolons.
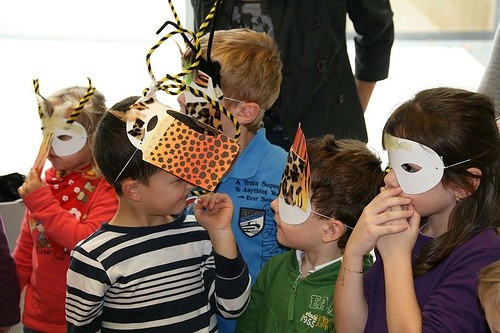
65;96;252;332
9;86;120;333
192;0;395;160
331;87;500;333
175;29;295;333
477;258;500;333
232;137;381;333
0;215;21;329
